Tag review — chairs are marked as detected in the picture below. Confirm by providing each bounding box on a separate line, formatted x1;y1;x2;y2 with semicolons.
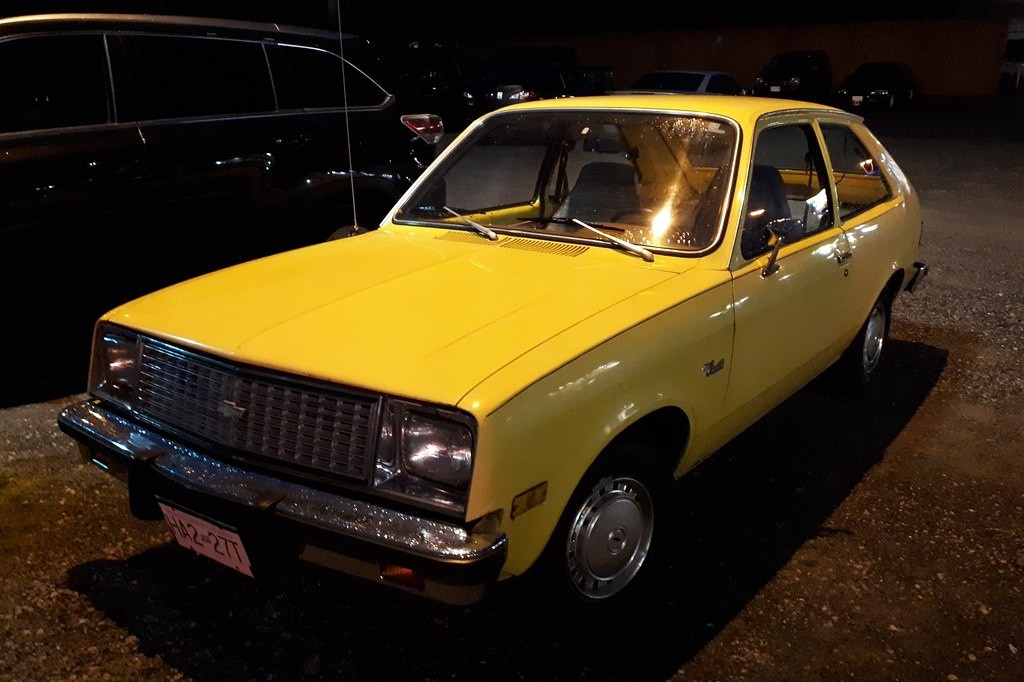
545;162;636;233
691;164;791;246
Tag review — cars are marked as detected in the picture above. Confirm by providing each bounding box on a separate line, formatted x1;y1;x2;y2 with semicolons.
402;49;926;142
57;91;929;625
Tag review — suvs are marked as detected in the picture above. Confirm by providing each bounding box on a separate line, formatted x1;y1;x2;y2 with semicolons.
0;13;450;340
